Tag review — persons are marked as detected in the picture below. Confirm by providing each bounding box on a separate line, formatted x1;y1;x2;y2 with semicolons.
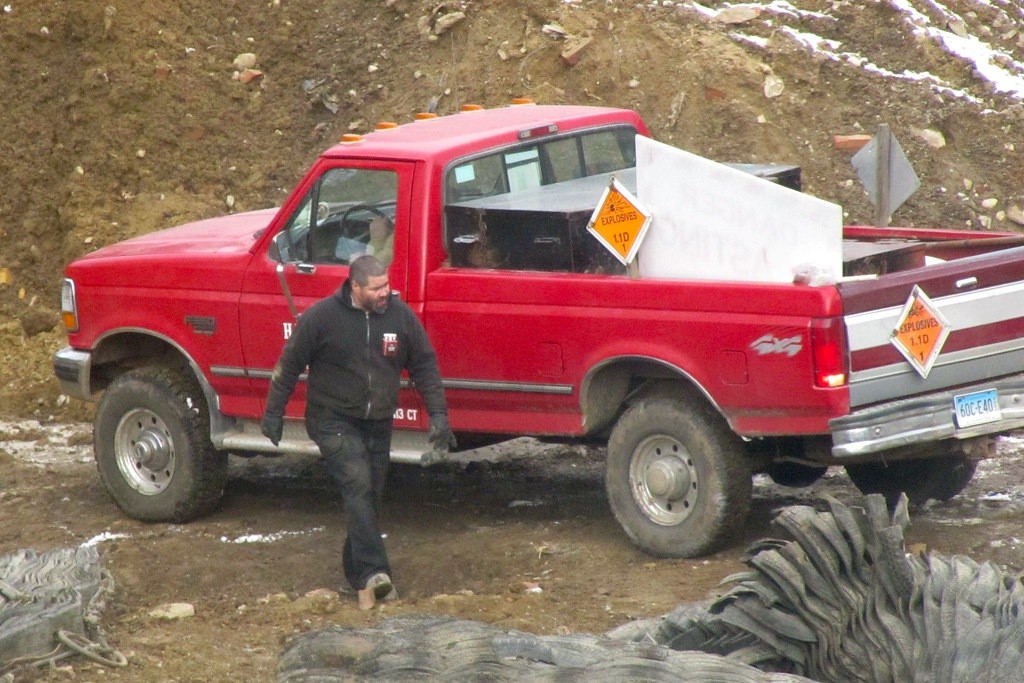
261;255;459;600
349;209;393;265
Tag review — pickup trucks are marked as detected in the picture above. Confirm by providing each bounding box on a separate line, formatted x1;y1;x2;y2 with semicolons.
53;97;1024;558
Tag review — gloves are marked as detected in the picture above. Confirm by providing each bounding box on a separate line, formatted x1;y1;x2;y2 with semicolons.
428;413;457;450
261;413;283;447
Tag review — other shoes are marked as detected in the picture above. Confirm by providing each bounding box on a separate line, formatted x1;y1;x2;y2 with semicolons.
357;588;377;611
366;573;392;601
